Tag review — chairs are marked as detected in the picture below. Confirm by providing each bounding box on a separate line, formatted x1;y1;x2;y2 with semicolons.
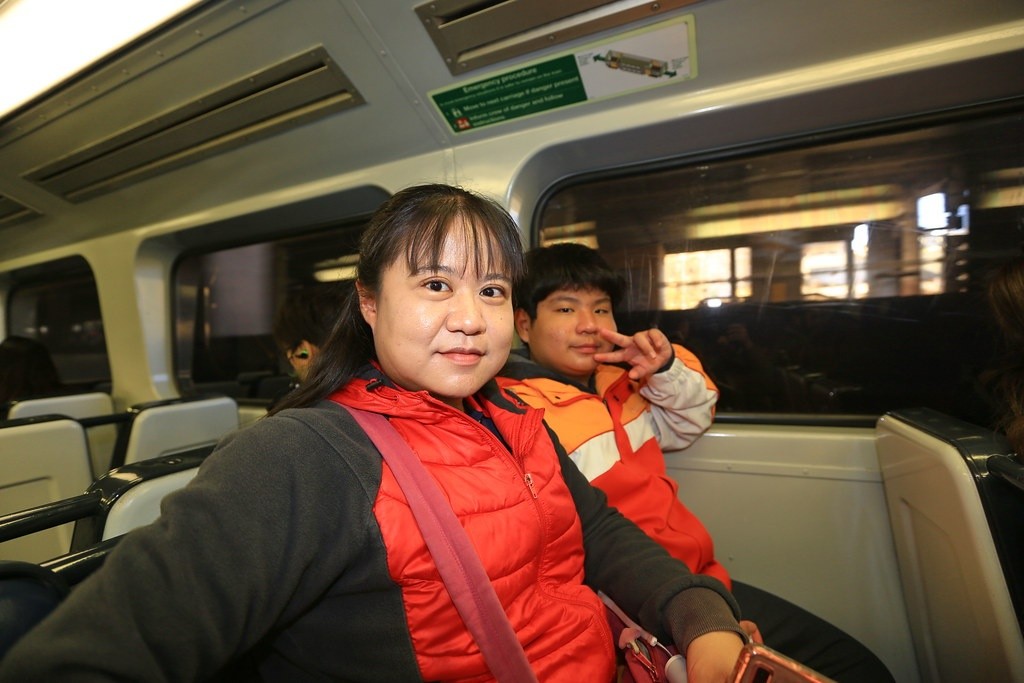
111;396;239;469
72;445;247;551
878;407;1024;683
0;414;96;563
0;391;119;477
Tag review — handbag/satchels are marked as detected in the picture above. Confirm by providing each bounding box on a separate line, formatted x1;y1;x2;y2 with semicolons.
624;636;684;683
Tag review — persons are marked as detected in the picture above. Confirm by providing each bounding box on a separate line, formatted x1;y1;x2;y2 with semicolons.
247;329;324;413
0;182;753;683
496;243;896;683
